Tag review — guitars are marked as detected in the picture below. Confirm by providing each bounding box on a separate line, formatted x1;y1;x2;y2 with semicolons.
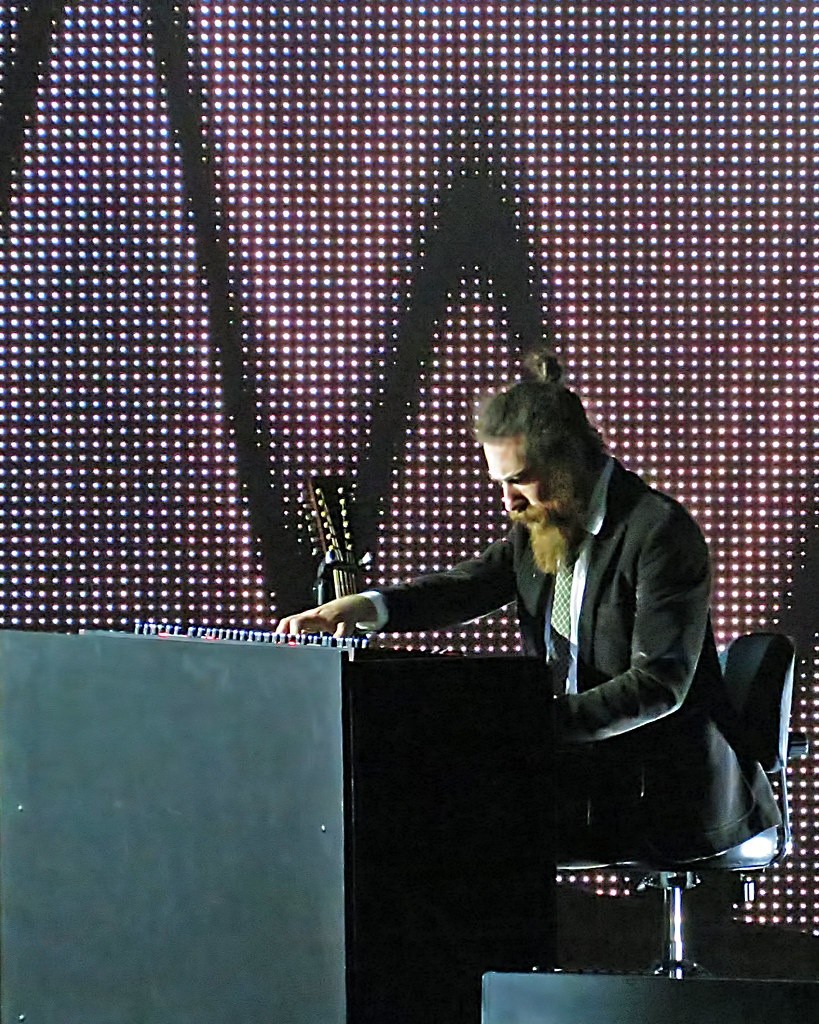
303;473;360;604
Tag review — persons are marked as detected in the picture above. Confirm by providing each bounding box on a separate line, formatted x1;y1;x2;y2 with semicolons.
273;347;784;971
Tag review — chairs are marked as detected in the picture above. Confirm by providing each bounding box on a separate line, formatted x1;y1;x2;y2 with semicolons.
557;629;810;978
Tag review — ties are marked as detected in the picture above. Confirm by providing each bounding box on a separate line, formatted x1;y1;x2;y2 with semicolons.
548;548;575;698
